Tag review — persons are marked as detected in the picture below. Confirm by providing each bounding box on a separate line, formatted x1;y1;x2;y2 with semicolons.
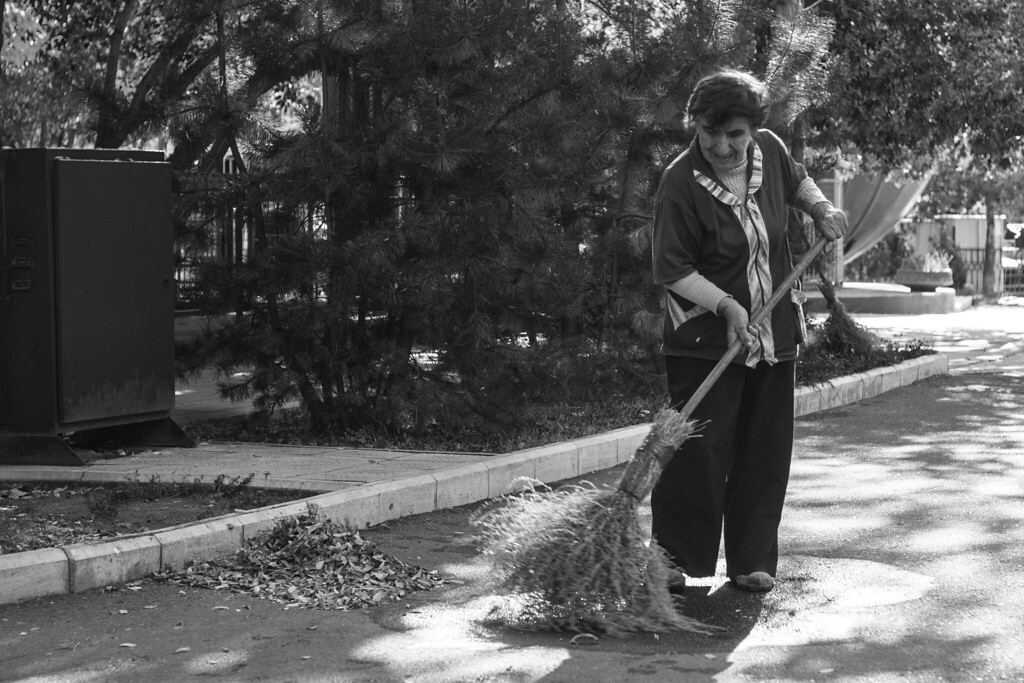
1015;228;1024;273
650;70;849;590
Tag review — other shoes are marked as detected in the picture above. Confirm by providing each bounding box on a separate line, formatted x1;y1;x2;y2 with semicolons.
665;568;685;589
730;570;774;592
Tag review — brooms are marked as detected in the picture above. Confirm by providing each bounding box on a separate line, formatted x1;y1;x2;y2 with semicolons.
469;235;829;636
